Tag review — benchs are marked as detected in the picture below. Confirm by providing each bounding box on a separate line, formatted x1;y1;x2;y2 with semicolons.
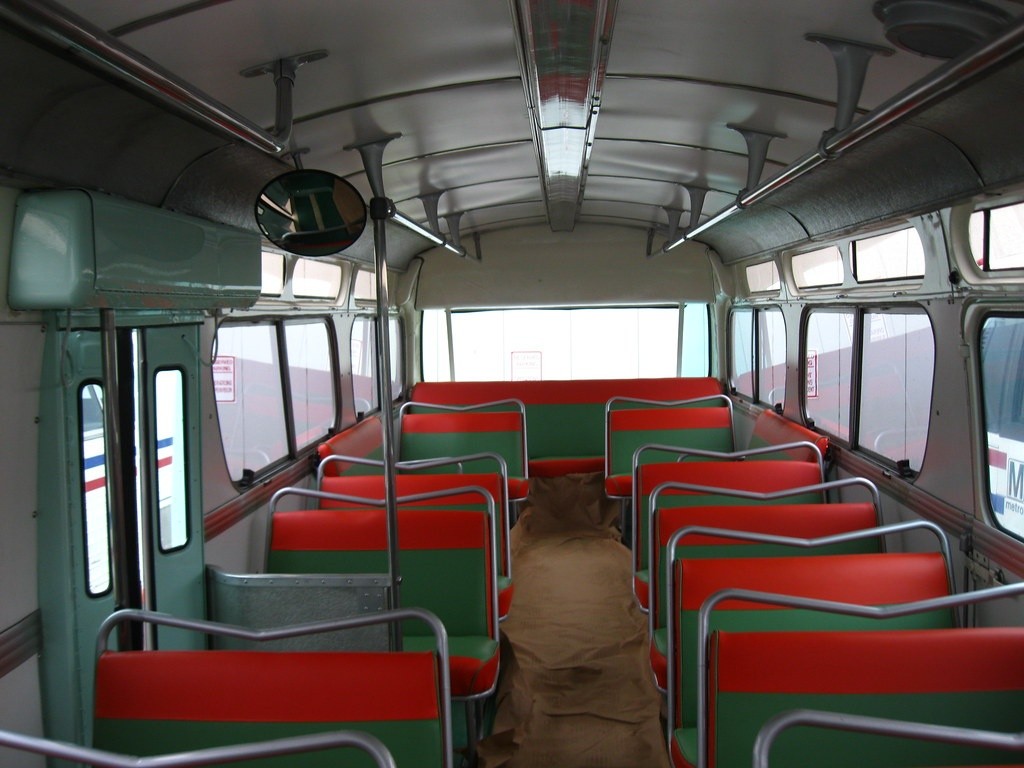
408;377;725;477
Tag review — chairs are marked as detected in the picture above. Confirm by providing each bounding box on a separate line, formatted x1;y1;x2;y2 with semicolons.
317;415;463;499
698;583;1024;768
256;487;499;767
750;708;1024;768
93;609;453;768
605;395;744;543
648;476;887;718
632;440;829;616
0;726;398;768
316;452;513;626
398;398;528;527
665;518;963;768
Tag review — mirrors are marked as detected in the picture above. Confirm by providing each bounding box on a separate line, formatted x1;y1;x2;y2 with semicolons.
253;166;369;258
